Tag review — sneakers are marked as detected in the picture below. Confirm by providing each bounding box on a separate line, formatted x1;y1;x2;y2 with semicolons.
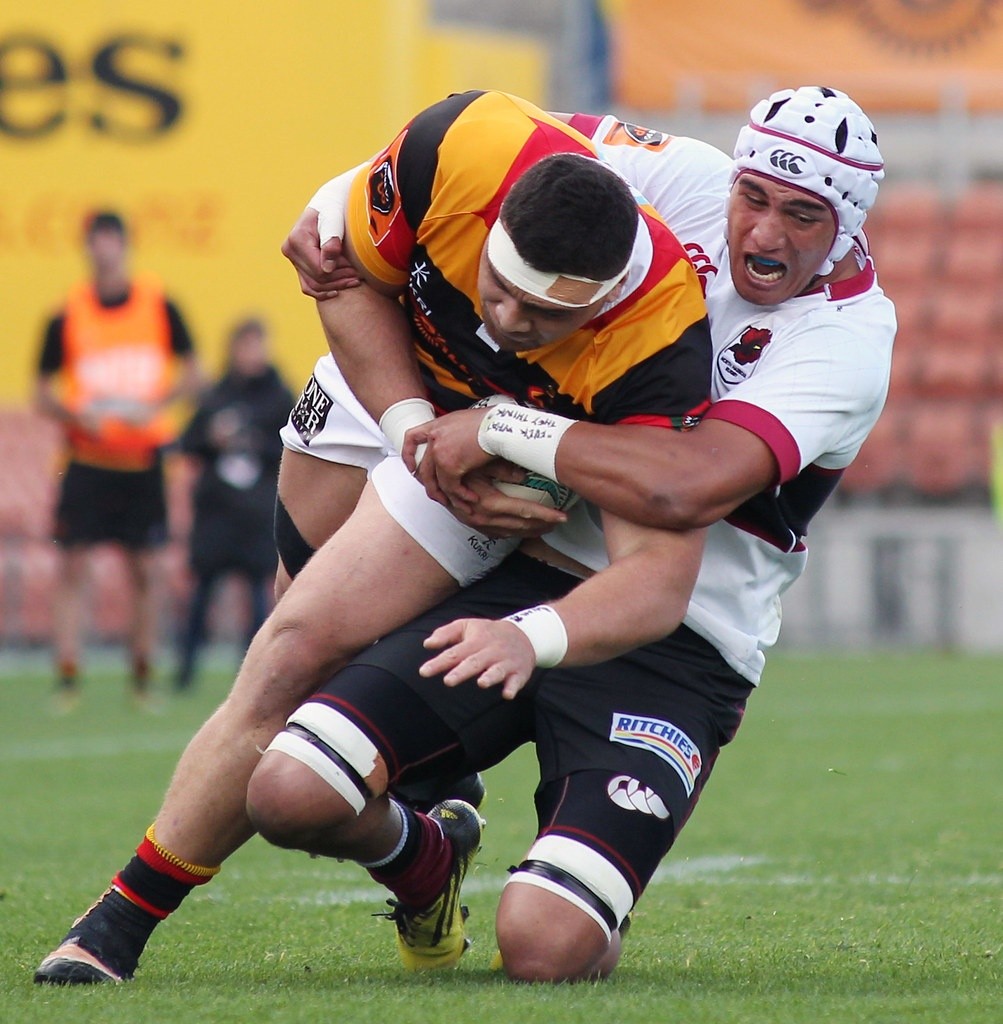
33;888;162;987
368;800;486;972
398;770;486;818
482;949;505;974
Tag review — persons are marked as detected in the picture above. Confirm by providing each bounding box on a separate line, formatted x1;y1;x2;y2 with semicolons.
32;86;900;985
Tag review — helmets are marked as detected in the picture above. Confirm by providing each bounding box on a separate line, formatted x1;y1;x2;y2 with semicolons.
729;86;886;277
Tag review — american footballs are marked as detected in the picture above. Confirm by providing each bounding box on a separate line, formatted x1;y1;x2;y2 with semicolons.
460;393;582;515
